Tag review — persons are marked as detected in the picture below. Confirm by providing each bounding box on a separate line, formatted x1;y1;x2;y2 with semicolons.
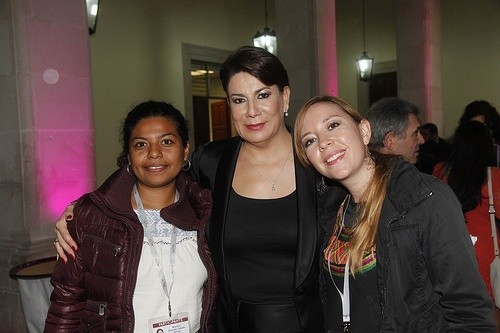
54;44;324;333
43;100;219;333
362;96;500;318
293;95;497;332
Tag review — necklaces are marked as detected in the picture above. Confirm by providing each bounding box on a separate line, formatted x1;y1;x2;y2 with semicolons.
243;149;292;191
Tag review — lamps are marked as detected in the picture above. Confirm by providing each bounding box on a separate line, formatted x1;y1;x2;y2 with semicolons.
85;0;100;35
355;0;374;81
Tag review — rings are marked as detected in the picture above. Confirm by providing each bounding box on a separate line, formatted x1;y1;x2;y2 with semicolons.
53;239;59;247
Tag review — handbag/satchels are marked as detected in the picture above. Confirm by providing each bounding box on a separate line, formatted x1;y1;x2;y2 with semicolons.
490;256;500;313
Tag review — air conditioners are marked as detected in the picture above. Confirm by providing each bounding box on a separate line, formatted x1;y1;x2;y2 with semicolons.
253;0;277;57
